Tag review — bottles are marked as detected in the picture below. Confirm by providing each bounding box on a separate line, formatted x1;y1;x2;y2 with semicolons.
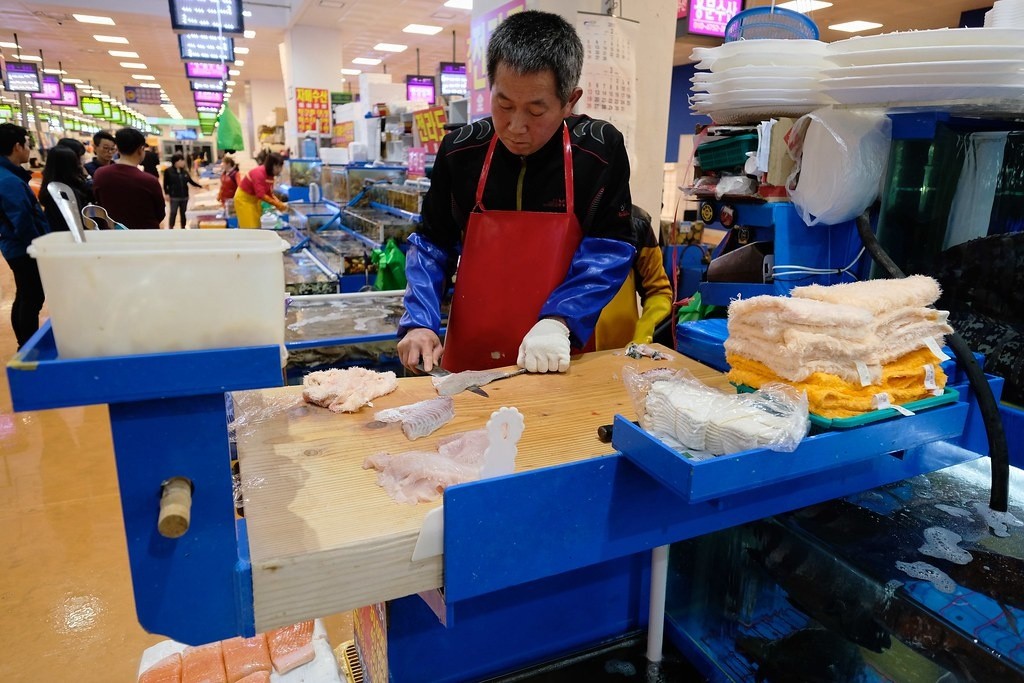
301;136;316;159
373;102;390;117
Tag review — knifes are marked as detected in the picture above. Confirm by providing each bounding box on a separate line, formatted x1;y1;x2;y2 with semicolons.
415;357;490;398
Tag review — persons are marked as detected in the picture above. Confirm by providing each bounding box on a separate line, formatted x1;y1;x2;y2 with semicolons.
217;151;284;229
569;204;674;354
163;154;205;230
397;11;638;374
0;110;209;350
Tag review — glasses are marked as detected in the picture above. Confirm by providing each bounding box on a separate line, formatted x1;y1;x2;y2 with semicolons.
103;146;118;154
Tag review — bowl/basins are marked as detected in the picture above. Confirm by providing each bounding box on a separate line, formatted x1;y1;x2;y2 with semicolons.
25;230;292;369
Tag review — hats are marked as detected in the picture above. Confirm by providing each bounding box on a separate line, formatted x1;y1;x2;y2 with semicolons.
114;127;150;148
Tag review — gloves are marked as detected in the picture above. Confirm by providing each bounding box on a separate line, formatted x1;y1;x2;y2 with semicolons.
516;319;570;373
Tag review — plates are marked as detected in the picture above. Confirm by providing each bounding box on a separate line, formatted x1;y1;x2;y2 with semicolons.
688;27;1023;122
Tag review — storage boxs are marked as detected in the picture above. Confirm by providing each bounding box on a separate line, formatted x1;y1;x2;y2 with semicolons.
695;131;760;172
26;226;291;374
663;455;1024;683
285;158;455;384
726;376;961;436
184;198;241;228
